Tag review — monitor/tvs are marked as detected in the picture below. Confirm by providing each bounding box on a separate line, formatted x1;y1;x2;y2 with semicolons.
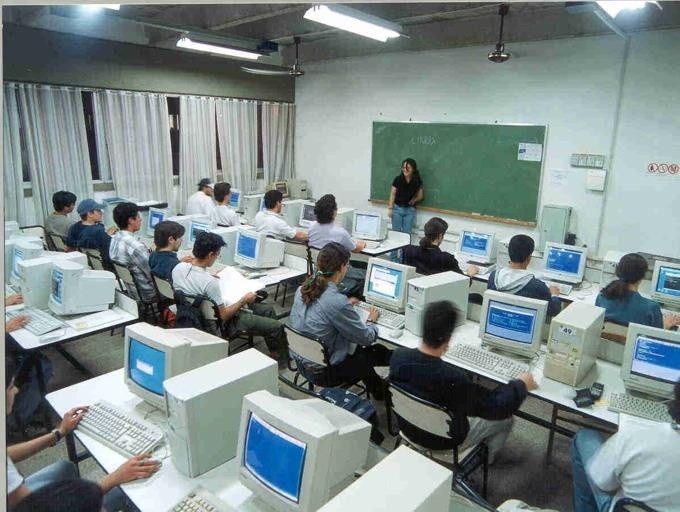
124;321;229;422
4;179;388;316
648;260;680;312
456;228;499;269
478;289;549;363
362;257;422;314
620;322;680;404
235;389;372;512
539;241;588;291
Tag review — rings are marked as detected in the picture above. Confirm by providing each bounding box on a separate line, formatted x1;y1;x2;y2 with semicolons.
139;461;144;466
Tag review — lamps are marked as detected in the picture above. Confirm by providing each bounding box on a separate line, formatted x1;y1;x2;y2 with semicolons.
175;31;272;60
302;5;411;44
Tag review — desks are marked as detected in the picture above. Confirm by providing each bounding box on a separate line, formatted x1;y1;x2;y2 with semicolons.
46;365;496;512
7;289;138;433
351;294;680;443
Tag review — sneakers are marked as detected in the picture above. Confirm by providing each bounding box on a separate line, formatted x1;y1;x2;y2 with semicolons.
7;424;46;440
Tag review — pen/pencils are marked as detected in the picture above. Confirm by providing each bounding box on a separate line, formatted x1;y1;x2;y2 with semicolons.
256;294;264;298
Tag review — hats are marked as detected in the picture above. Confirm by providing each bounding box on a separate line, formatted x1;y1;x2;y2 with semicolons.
77;199;106;215
199;178;213;188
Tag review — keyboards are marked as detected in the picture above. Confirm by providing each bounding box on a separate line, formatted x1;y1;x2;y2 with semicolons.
459;263;488;275
607;391;676;424
540;280;572;295
167;483;237;512
442;341;530;382
74;399;164;460
10;307;64;336
353;300;406;330
659;309;680;327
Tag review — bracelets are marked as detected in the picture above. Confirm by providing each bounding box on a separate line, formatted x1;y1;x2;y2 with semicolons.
388;208;394;210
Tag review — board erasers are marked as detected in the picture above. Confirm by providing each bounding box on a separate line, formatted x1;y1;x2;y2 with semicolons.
472;212;481;217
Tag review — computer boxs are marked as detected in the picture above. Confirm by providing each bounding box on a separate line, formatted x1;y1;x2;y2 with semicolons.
315;444;454;512
543;300;606;387
497;235;513;267
405;270;470;337
600;251;623;289
163;347;279;478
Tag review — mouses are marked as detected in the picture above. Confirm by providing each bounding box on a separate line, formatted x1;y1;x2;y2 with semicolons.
146;457;163;467
388;328;403;339
576;294;585;300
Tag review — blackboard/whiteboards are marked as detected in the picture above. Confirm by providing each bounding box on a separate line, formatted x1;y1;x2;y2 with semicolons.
367;121;549;227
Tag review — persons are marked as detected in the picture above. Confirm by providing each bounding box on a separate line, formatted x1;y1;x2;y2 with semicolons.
11;479;104;512
290;240;394;401
183;178;216;220
5;287;50;440
252;189;308;244
6;357;162;511
390;298;538;497
308;195;366;297
210;181;241;227
594;252;680;329
44;190;291;363
570;380;679;511
387;158;424;232
407;218;467;276
486;234;561;316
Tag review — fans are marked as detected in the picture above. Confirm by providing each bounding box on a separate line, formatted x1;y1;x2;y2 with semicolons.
487;3;520;62
238;36;307;77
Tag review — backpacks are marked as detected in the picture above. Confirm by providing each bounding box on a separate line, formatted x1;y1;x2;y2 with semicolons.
173;290;210;332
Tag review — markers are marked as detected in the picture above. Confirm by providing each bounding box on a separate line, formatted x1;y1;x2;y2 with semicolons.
506;218;518;222
483;215;495;218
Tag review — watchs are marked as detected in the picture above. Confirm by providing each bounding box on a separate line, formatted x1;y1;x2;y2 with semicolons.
51;428;63;445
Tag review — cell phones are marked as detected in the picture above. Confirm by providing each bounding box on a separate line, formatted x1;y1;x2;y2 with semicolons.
590;383;604;401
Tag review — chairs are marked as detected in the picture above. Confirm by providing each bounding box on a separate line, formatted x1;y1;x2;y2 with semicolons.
382;380;492;502
4;214;679;373
281;323;388;403
